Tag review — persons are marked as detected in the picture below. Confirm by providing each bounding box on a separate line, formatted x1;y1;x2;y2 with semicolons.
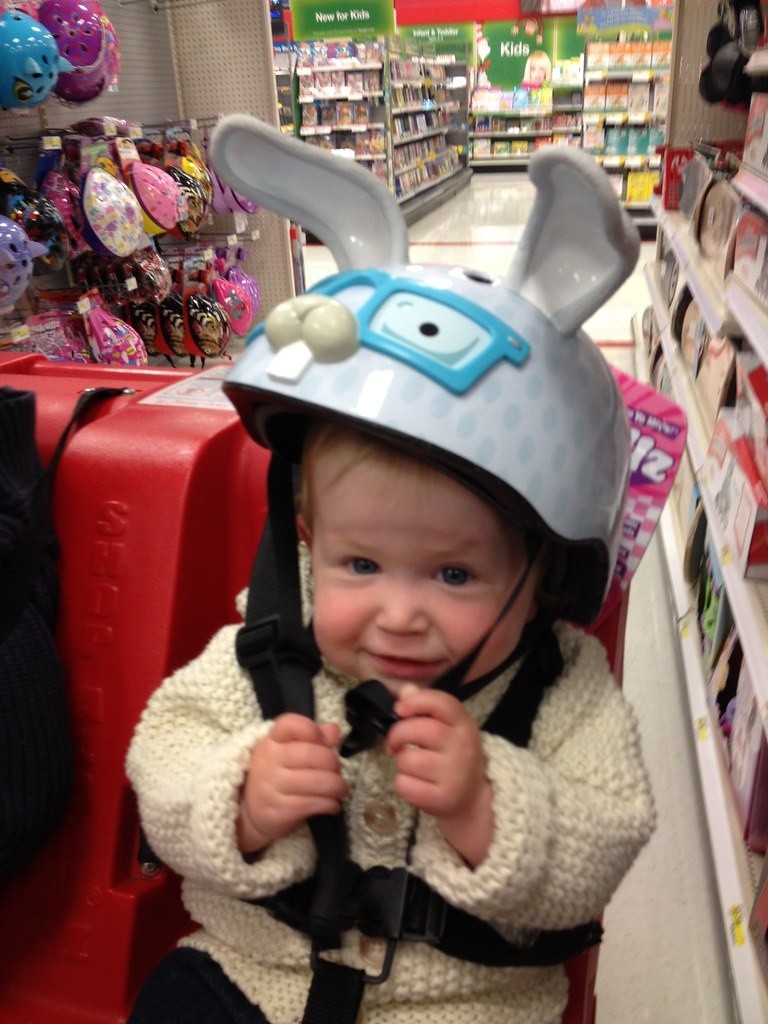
126;115;659;1024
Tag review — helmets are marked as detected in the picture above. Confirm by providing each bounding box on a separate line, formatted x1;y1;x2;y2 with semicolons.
38;0;118;109
0;7;76;116
0;127;259;371
209;113;641;627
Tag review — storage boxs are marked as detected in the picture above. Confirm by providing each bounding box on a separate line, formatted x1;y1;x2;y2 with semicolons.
584;41;671;202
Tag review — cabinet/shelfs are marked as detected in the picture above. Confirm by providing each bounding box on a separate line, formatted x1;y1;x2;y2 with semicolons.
582;41;671;224
471;85;582;166
632;0;768;1024
270;22;473;247
